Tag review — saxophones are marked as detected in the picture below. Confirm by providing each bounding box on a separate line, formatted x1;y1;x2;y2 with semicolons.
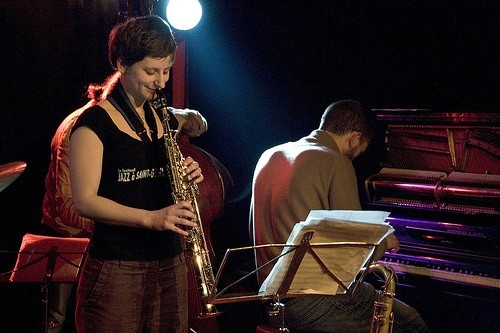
360;264;398;333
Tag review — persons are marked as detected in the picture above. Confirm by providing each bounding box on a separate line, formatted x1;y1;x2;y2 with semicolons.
38;71;121;333
68;15;204;333
249;100;429;333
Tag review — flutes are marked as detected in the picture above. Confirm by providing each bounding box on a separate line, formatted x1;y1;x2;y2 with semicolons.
155;88;223;319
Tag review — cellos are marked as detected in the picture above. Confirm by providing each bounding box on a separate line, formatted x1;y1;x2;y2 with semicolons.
75;35;236;333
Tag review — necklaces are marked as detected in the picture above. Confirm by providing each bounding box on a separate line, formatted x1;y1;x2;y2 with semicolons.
108;79;160;160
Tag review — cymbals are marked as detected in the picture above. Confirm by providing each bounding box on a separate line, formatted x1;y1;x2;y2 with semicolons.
0;161;27;193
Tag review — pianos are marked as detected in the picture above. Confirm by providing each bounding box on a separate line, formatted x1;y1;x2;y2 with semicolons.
369;217;500;303
